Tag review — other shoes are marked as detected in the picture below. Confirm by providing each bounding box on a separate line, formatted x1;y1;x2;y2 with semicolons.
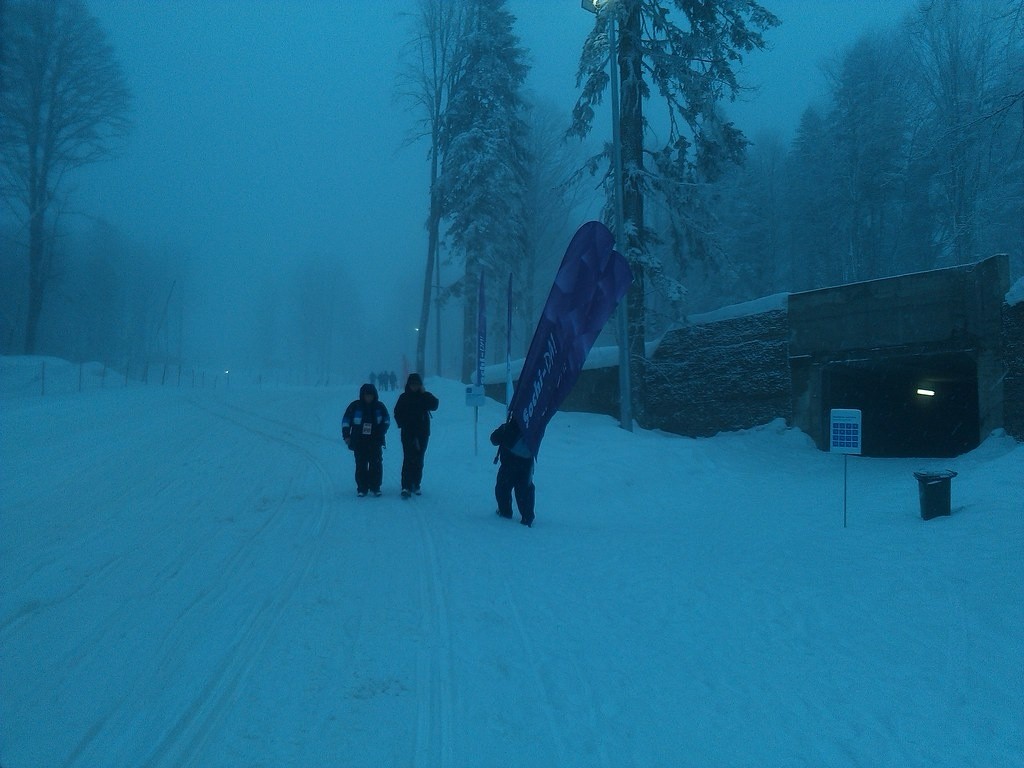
401;489;411;497
496;508;512;519
357;492;364;497
521;520;533;527
414;489;421;495
371;491;381;496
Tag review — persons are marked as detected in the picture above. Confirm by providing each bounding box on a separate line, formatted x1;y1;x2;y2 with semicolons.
367;371;398;391
393;373;439;499
342;384;391;497
491;413;536;527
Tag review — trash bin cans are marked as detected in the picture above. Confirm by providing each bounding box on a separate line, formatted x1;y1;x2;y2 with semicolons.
911;468;958;520
